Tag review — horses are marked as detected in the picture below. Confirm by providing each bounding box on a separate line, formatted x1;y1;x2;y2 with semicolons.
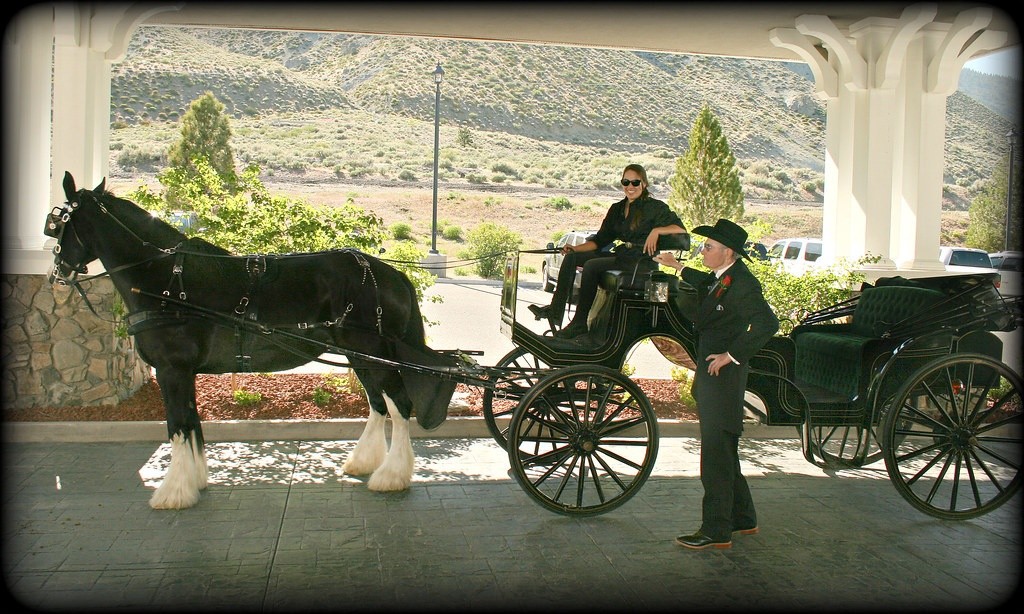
48;170;426;509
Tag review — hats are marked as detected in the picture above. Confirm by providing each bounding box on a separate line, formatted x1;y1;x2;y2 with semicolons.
691;218;754;264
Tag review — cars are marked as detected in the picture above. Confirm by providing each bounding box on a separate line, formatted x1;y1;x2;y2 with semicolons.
939;246;1002;294
987;250;1020;298
689;241;772;266
767;238;823;279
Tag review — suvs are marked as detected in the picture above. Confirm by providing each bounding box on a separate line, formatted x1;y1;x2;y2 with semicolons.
541;230;615;305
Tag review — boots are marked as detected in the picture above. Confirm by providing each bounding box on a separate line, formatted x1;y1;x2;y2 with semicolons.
555;306;591;337
527;293;567;326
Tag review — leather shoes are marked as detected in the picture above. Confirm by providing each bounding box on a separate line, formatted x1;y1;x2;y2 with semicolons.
732;522;758;534
677;529;732;550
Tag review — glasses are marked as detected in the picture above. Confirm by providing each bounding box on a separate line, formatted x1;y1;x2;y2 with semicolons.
704;243;714;251
621;179;641;186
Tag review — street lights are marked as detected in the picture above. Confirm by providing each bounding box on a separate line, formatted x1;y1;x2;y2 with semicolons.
428;61;445;254
1005;128;1018;251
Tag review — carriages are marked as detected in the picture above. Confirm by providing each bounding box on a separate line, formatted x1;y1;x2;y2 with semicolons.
43;170;1020;520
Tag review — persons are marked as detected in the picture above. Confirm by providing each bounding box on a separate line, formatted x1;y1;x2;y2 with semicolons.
652;219;779;549
528;164;687;339
